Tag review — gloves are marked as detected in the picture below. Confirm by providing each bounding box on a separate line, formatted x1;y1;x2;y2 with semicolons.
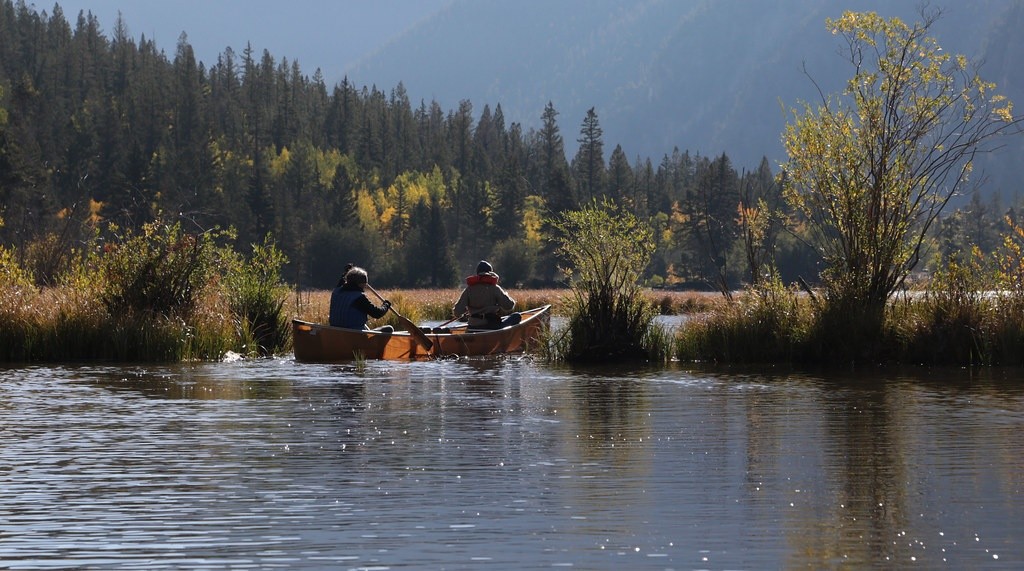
383;299;391;307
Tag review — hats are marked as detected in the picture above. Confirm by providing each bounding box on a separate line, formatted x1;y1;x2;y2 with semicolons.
476;261;491;274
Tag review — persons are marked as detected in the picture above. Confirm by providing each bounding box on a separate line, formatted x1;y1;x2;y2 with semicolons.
330;263;394;333
453;261;522;333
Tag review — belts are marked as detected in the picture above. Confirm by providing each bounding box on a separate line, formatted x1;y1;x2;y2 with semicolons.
470;313;496;319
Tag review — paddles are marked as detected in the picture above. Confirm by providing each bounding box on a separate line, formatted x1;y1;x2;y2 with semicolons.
430;309;470;333
347;260;435;352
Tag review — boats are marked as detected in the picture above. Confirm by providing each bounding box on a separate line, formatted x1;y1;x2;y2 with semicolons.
292;304;552;364
